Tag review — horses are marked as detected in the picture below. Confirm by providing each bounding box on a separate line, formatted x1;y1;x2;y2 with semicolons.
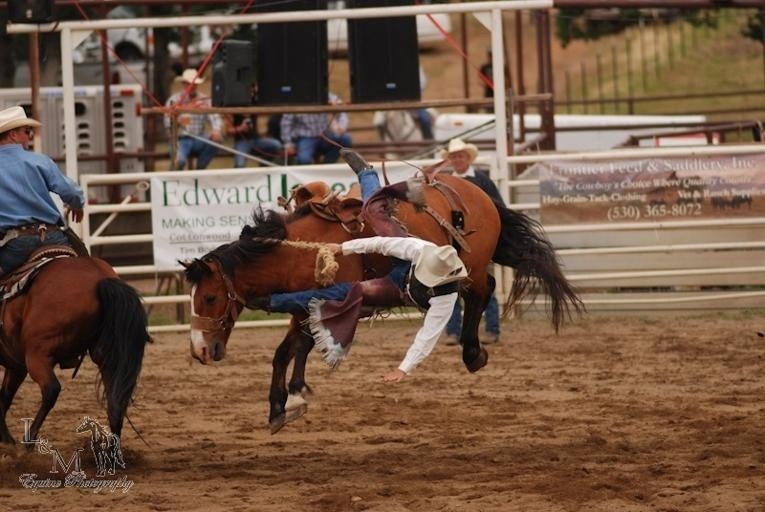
176;173;587;435
0;256;155;453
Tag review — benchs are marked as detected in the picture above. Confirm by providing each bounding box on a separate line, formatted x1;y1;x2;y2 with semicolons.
150;141;496;161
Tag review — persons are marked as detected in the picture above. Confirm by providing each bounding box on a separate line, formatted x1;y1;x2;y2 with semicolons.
164;68;223;170
246;236;473;383
413;65;434;139
225;81;353;168
440;137;508;348
0;106;85;276
480;48;512;97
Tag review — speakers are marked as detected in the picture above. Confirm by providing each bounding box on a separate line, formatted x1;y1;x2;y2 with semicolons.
211;40;255;107
345;0;421;104
254;0;329;107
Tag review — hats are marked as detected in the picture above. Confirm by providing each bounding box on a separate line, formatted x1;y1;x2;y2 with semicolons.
413;244;468;288
0;106;43;134
440;137;478;163
174;68;206;85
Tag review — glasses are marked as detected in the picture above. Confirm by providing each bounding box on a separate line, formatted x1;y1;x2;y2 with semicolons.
19;126;33;135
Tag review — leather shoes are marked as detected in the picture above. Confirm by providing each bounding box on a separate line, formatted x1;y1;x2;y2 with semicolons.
338;148;370;173
479;333;500;345
248;296;271;310
446;334;459;344
